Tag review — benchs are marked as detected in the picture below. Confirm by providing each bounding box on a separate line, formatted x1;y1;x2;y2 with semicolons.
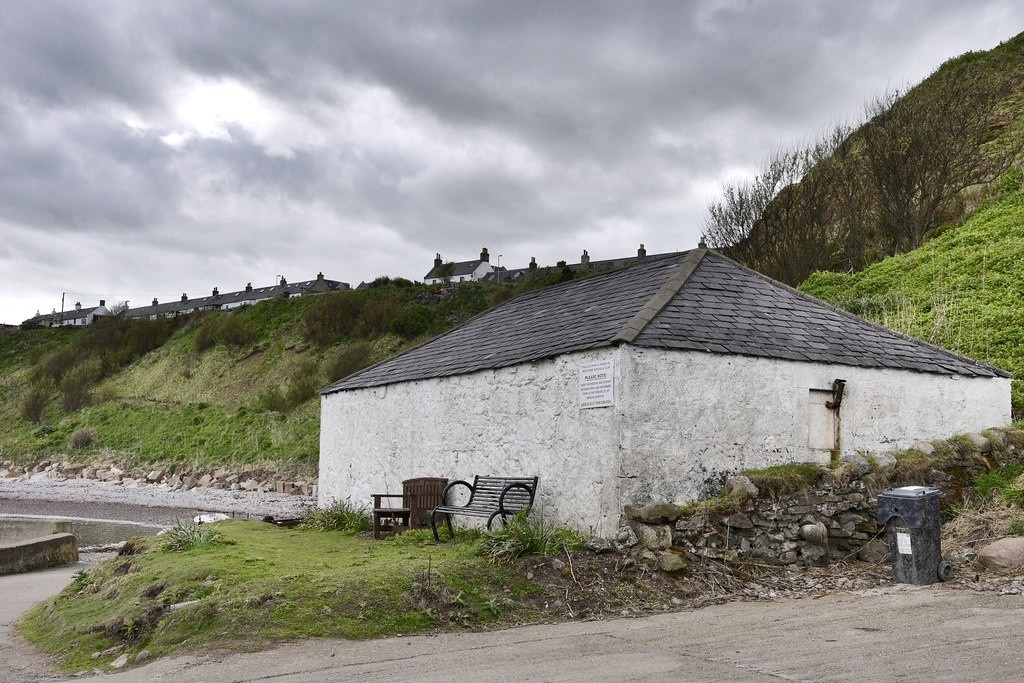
370;477;449;541
429;474;540;544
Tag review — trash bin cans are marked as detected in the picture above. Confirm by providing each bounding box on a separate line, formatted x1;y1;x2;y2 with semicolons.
877;486;952;586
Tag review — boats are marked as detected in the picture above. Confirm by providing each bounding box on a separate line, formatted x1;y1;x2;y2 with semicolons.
193;512;230;525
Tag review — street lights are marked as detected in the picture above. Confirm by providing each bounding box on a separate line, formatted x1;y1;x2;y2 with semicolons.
125;300;130;319
497;254;502;284
275;275;281;297
